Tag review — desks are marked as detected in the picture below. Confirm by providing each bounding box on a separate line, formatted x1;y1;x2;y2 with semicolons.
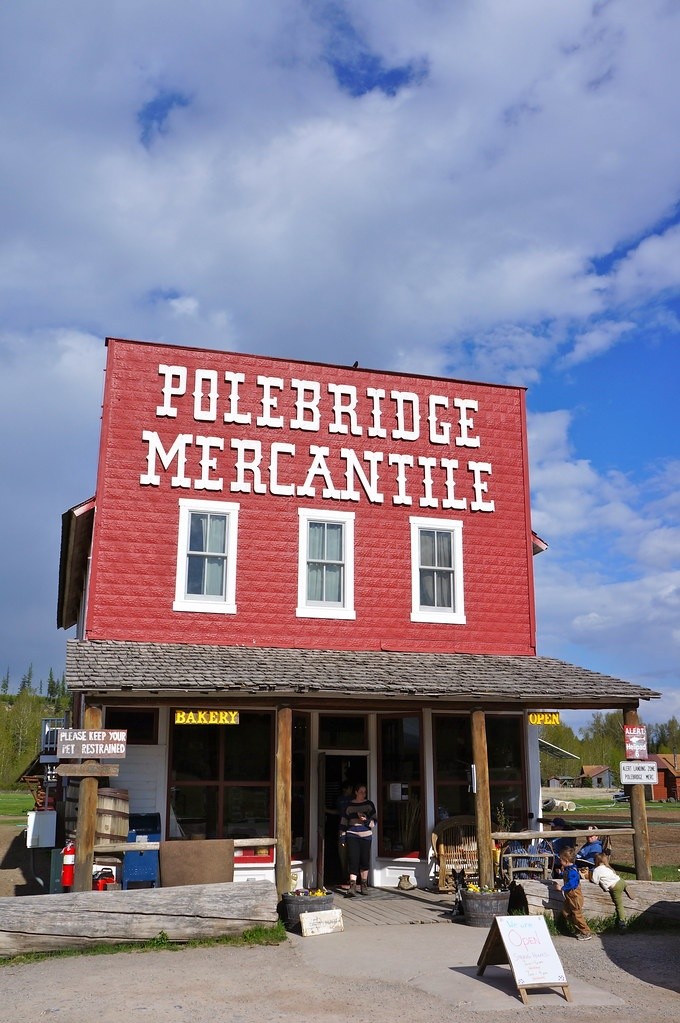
505;852;555;884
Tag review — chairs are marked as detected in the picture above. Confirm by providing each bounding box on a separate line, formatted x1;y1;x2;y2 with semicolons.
576;835;613;879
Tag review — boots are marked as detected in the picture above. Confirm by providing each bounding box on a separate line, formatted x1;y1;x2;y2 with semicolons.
360;878;368;895
346;880;357;897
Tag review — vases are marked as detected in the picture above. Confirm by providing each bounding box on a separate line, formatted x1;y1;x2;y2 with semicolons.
460;890;510;927
283;893;334;933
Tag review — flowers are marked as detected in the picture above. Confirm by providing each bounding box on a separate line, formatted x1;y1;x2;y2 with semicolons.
466;882;503;892
288;888;327;896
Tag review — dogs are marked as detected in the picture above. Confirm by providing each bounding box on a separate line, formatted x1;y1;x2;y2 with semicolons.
451;868;468;916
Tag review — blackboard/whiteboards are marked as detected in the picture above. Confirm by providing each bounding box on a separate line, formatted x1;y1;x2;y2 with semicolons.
478;915;568;988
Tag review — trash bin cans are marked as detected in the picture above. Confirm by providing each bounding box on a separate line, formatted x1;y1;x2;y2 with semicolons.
122;812;161;890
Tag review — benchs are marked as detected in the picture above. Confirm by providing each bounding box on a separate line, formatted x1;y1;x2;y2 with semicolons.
431;813;498;891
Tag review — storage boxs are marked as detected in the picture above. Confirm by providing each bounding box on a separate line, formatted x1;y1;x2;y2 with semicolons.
299;908;343;936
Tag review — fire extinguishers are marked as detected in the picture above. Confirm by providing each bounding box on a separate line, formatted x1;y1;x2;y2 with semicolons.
59;842;75;887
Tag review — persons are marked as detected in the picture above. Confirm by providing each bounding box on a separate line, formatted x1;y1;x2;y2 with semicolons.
538;818;603;878
589;853;634;934
292;790;304;851
324;781;376;897
554;850;592;940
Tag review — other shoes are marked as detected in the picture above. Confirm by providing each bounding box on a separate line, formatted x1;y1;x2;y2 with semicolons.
626;886;635;900
615;919;626;930
575;932;592;941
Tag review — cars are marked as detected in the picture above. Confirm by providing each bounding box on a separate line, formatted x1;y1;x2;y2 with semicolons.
612;789;631;802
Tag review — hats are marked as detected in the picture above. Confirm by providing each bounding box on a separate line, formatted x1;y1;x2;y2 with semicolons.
587;825;598;830
548;817;566;827
